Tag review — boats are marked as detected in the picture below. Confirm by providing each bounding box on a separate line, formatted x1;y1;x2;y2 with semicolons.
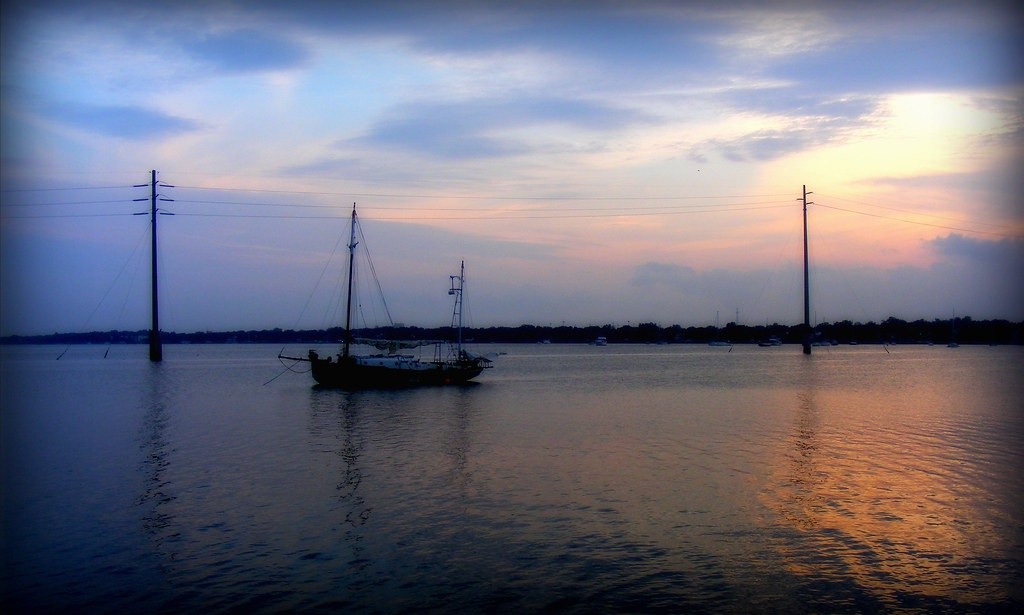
813;340;857;346
948;343;959;347
760;339;782;347
278;200;492;387
710;341;731;347
596;337;607;346
